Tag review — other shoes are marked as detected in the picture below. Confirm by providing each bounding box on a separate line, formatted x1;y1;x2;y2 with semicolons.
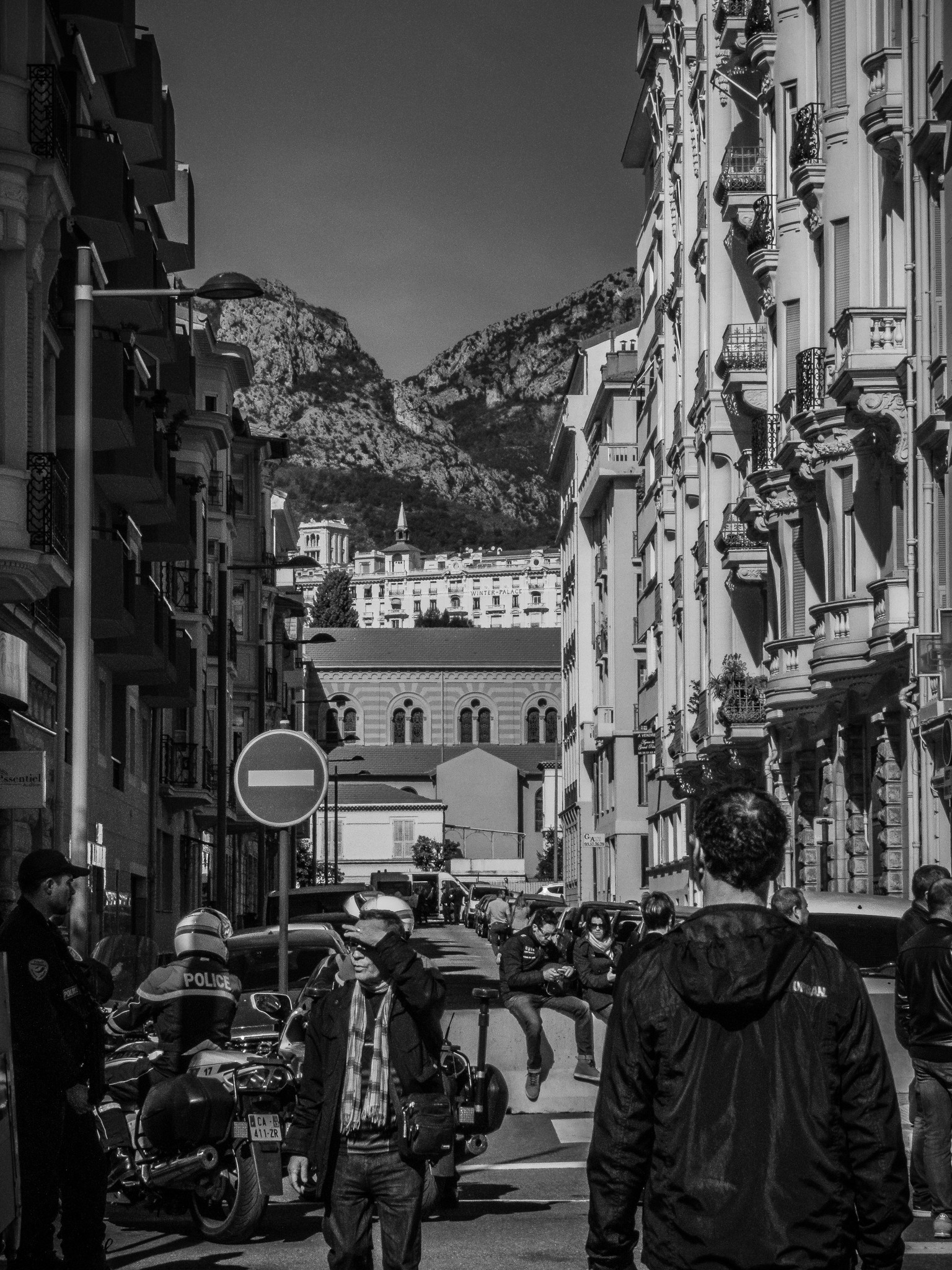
525;1072;541;1100
573;1059;601;1081
912;1200;932;1217
15;1238;113;1270
933;1212;952;1237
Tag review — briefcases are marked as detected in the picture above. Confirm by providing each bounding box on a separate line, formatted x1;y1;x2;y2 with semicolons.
496;953;501;965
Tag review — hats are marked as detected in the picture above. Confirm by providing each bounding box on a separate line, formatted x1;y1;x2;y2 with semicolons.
18;849;92;891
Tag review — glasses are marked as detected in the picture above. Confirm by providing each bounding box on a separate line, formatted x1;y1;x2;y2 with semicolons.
538;927;556;937
591;923;604;930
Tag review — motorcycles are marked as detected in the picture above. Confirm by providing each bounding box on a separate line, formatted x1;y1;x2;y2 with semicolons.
89;936;508;1246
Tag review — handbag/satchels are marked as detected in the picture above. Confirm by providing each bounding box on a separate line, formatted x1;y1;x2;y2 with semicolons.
397;1090;453;1163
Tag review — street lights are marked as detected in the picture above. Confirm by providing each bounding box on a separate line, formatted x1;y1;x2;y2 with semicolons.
323;754;366;884
71;250;266;962
214;552;322;915
265;632;336;889
311;734;363;883
332;765;371;885
287;688;353;888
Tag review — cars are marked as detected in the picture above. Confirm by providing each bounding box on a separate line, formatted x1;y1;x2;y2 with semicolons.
462;883;701;964
800;888;912;978
227;923;351;1043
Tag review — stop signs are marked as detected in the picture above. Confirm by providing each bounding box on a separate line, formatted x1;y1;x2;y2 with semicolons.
234;728;332;828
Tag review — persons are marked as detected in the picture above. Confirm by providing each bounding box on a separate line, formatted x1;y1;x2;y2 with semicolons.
612;891;675;1000
390;886;437;926
588;782;916;1269
93;908;242;1189
499;908;601;1099
486;889;512;964
573;907;623;1020
283;909;460;1270
440;887;453;925
511;896;530;934
771;888;839;950
293;891;446;1012
895;864;952;1237
444;885;465;925
5;849;113;1270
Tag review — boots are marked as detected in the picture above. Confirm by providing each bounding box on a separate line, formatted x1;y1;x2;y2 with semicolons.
107;1146;136;1193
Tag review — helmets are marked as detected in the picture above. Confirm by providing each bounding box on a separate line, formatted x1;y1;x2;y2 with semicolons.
343;892;384;920
361;895;414;937
173;912;229;964
539;962;563;995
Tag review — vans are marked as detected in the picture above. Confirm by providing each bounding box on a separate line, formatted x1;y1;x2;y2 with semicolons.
370;868;469;920
